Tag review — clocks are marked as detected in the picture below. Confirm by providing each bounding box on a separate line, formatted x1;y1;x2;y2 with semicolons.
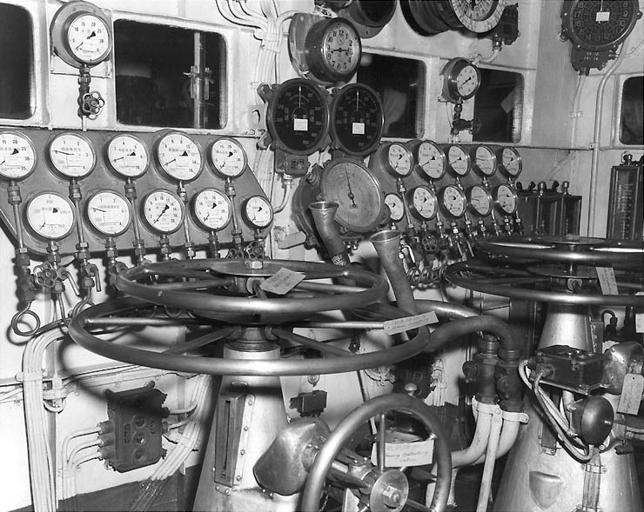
305;17;363;84
